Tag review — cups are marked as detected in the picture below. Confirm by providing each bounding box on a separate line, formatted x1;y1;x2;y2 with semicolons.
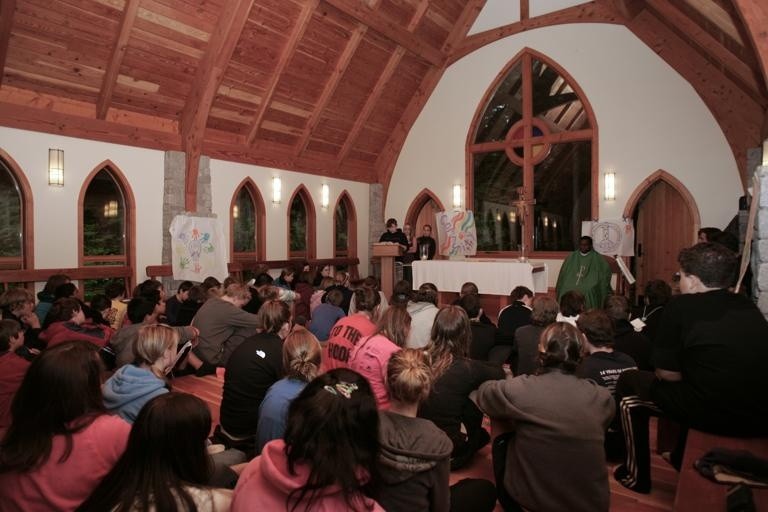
517;244;529;263
419;244;430;260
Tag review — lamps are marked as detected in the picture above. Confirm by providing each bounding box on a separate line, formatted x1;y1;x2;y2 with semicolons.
272;175;281;205
603;172;616;202
49;149;64;185
452;184;461;208
321;184;330;209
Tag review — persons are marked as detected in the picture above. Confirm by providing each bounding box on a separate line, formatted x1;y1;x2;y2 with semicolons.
418;306;490;474
696;227;724;243
0;339;135;510
82;391;233;511
367;348;496;511
213;300;292;455
403;223;418;280
379;218;408;279
609;244;764;494
415;225;437;260
231;368;384;512
3;266;722;418
556;236;610;309
468;323;617;512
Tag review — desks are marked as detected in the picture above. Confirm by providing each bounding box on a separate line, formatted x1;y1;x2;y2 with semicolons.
413;260;546;317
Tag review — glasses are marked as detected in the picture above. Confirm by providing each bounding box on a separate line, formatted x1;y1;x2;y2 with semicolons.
672;272;690;283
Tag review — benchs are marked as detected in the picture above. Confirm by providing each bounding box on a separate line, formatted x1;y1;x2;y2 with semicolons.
674;429;768;512
